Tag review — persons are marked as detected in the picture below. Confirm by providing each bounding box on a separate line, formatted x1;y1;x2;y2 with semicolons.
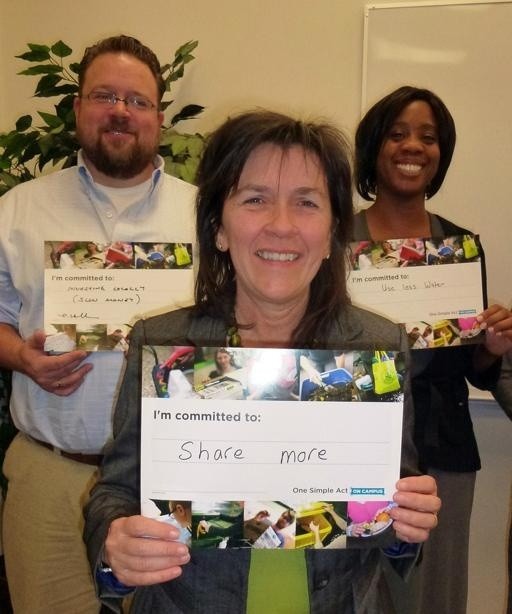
309;505;347;549
406;323;437;349
63;324;77;345
82;242;174;268
210;346;243;377
109;326;129;351
426;237;467;264
0;33;210;614
82;105;443;614
342;499;399;538
166;500;192;530
379;240;398;259
459;317;485;340
244;506;296;548
297;350;348;388
438;322;461;346
342;84;511;612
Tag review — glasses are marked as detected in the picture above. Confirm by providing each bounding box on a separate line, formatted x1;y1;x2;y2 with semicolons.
169;507;178;519
80;90;160;111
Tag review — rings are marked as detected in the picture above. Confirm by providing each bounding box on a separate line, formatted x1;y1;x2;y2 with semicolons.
55;382;64;390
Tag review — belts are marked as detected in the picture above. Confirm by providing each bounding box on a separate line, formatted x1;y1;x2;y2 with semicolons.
28;434;105;467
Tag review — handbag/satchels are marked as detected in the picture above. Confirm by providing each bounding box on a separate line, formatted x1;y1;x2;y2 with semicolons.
371;360;400;394
175;247;191;266
462;239;479;259
